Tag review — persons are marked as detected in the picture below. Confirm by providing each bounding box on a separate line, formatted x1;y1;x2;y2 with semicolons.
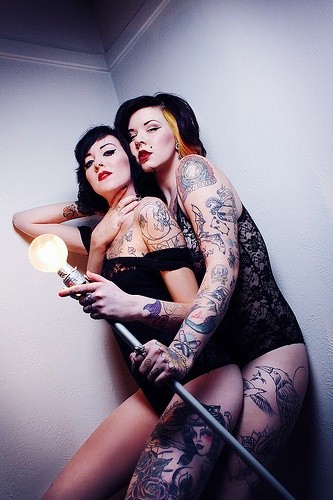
12;125;244;500
115;91;310;500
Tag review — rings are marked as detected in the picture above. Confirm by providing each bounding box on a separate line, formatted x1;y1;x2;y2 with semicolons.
134;345;148;358
86;293;94;303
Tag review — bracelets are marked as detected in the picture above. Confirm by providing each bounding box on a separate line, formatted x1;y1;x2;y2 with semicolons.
172;339;198;362
168;347;189;376
